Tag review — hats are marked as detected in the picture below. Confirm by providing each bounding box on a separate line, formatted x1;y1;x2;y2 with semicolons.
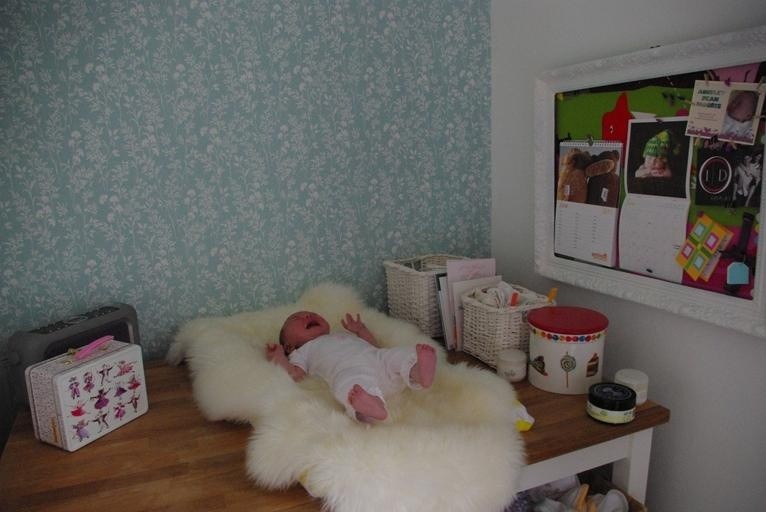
643;130;680;160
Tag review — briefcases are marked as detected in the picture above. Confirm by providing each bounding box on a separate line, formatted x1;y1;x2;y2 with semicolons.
23;334;150;453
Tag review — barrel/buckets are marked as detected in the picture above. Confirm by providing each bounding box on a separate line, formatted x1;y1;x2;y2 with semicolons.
526;304;610;395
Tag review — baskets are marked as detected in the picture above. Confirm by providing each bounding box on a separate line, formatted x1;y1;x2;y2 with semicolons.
457;282;556;372
383;253;472;339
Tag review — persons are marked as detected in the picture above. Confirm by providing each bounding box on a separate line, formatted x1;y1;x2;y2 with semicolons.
634;127;674;179
694;136;762;208
265;310;437;425
721;90;757;140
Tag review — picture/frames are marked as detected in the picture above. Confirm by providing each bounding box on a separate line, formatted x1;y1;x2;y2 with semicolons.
534;25;766;338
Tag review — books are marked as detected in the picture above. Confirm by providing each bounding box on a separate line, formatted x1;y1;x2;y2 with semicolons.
434;256;504;353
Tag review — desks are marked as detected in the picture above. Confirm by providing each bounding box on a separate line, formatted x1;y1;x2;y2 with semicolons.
0;336;670;512
